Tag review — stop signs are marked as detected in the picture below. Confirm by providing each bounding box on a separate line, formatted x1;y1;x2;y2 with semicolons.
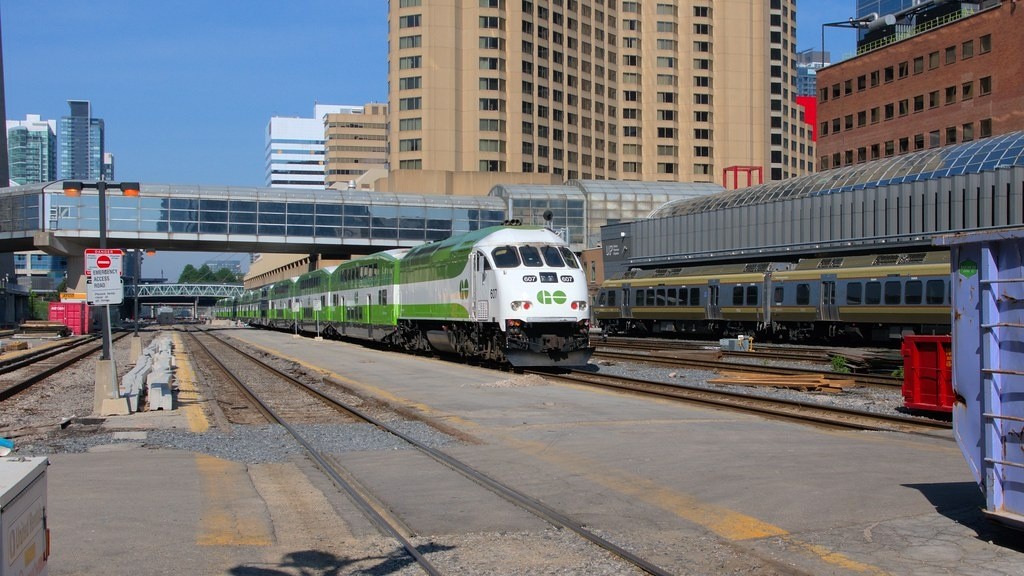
97;256;112;270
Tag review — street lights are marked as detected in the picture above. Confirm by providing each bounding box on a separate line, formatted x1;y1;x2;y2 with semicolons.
62;180;141;358
121;247;156;338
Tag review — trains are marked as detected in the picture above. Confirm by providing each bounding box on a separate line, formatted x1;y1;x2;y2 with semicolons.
590;262;953;346
213;209;607;373
